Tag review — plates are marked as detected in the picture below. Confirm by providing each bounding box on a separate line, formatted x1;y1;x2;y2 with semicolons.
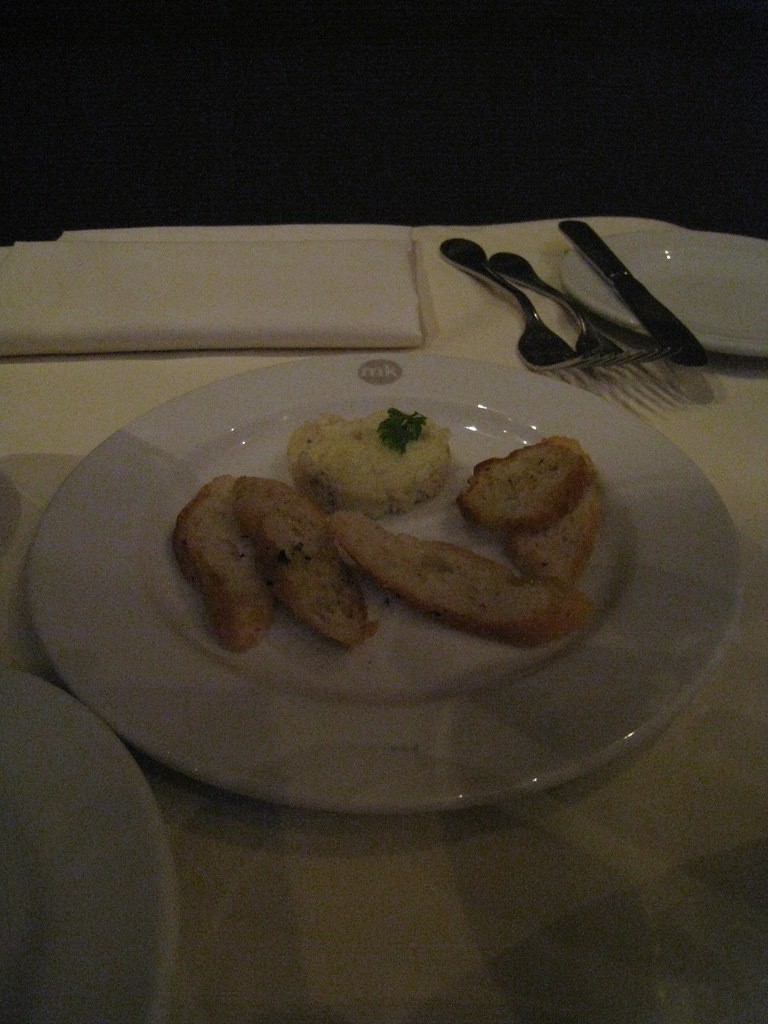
22;349;740;818
0;669;182;1024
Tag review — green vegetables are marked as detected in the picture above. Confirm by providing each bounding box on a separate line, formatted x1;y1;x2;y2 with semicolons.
377;406;428;455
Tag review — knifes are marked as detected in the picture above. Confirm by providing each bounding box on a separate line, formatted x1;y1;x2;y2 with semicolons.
559;220;709;367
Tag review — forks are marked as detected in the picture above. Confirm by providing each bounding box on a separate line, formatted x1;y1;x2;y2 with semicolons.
487;251;644;369
438;238;616;372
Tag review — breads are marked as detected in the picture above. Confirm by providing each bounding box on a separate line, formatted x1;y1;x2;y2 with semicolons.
232;476;368;647
454;435;594;533
173;474;277;652
328;511;591;648
